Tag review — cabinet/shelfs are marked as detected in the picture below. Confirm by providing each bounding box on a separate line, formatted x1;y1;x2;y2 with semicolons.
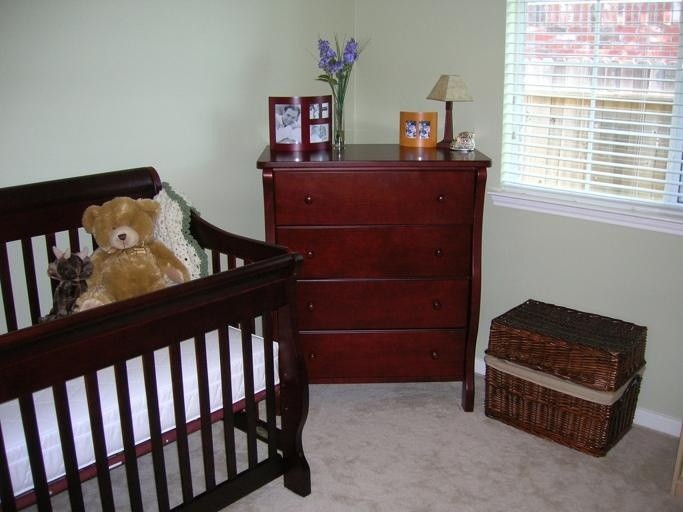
257;144;492;409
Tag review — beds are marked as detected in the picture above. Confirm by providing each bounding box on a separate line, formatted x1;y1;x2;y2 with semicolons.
0;164;312;511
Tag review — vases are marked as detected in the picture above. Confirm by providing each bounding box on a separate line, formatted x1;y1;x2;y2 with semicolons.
326;96;347;153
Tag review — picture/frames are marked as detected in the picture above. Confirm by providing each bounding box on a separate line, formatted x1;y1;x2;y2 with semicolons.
264;96;330;153
398;111;437;149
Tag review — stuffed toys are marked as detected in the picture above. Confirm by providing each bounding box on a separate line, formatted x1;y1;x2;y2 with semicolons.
74;196;190;314
36;246;93;323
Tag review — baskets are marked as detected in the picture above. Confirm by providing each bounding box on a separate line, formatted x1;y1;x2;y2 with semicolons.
484;298;649;394
481;364;643;461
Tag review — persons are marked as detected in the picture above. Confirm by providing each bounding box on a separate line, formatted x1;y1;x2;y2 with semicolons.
274;106;300;143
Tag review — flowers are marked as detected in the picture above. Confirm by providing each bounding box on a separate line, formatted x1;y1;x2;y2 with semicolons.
309;32;371;102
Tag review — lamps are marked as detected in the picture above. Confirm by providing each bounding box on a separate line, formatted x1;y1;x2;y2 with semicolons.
428;72;474;149
